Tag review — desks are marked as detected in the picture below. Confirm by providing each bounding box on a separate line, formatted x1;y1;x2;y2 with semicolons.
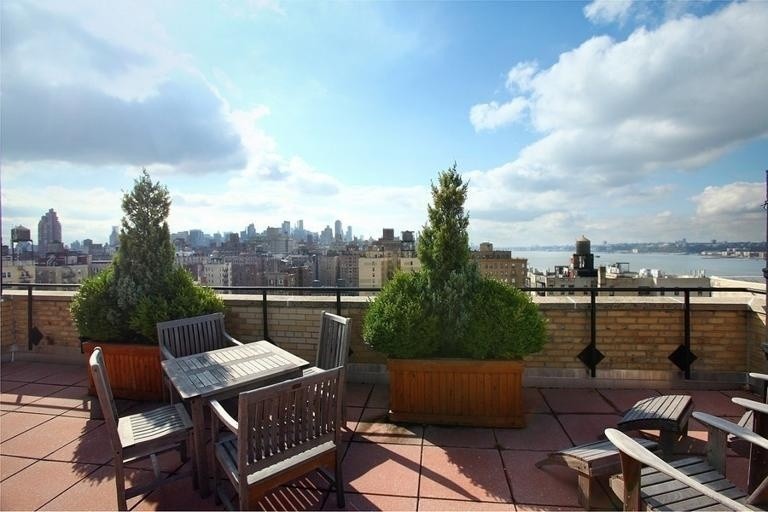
161;340;310;499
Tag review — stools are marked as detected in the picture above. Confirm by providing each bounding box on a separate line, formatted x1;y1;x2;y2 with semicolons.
619;395;695;460
536;439;661;510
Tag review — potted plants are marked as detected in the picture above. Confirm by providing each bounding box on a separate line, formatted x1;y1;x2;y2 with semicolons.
69;166;228;402
362;160;550;429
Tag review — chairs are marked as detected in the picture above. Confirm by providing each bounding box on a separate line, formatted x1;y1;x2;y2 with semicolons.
304;310;352;429
89;346;206;512
156;312;244;405
213;365;347;512
730;372;768;493
604;411;768;512
